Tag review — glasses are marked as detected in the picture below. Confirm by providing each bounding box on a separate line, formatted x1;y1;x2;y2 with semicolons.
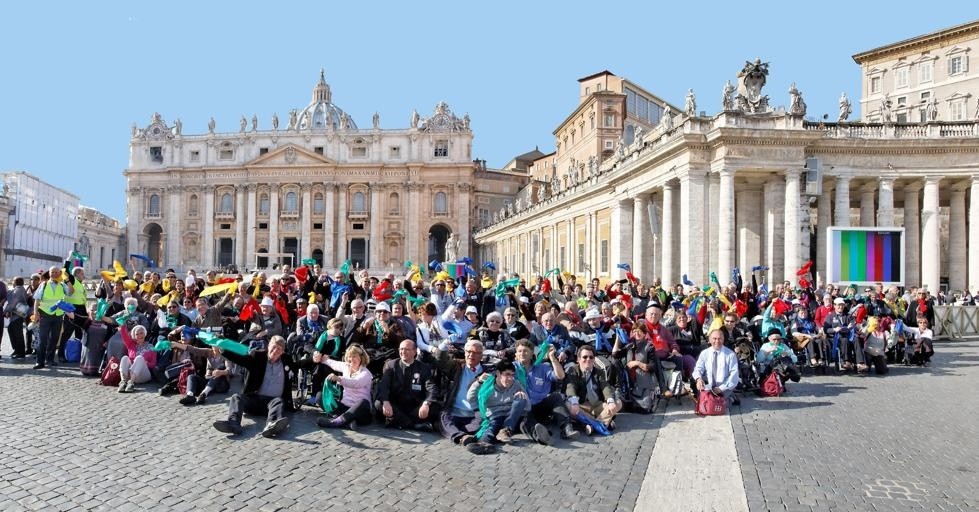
580;355;596;360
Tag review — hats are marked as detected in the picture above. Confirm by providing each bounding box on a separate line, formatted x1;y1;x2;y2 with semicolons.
259;265;858;338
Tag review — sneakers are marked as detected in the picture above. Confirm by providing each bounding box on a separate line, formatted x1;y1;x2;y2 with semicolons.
533;423;551;444
461;434;477;445
346;420;358;431
805;358;931;375
466;440;494;455
11;348;211;407
261;417;289;437
560;425;580;439
213;420;242;434
608;420;617;431
585;425;595;436
316;418;338;428
496;427;514;444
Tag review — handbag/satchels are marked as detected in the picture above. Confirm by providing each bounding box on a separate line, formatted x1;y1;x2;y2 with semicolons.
1;299;30;320
696;390;727;416
762;371;783;396
654;360;684;397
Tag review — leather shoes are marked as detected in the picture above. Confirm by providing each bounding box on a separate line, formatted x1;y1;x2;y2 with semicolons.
415;419;434;434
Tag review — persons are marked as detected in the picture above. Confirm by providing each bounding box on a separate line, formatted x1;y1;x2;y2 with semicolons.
373;111;380;128
173;117;183;135
410;108;420;128
445;233;461;263
839;92;852;121
722;79;739;111
924;91;939;121
879;92;893;122
462;110;470;127
207;116;216;133
478;87;695;228
789;81;800;114
239;108;350;130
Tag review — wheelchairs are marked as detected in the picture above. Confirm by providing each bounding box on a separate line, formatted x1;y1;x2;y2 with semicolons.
710;321;864;397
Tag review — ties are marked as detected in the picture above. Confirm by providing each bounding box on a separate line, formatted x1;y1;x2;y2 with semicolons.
711;352;717;387
